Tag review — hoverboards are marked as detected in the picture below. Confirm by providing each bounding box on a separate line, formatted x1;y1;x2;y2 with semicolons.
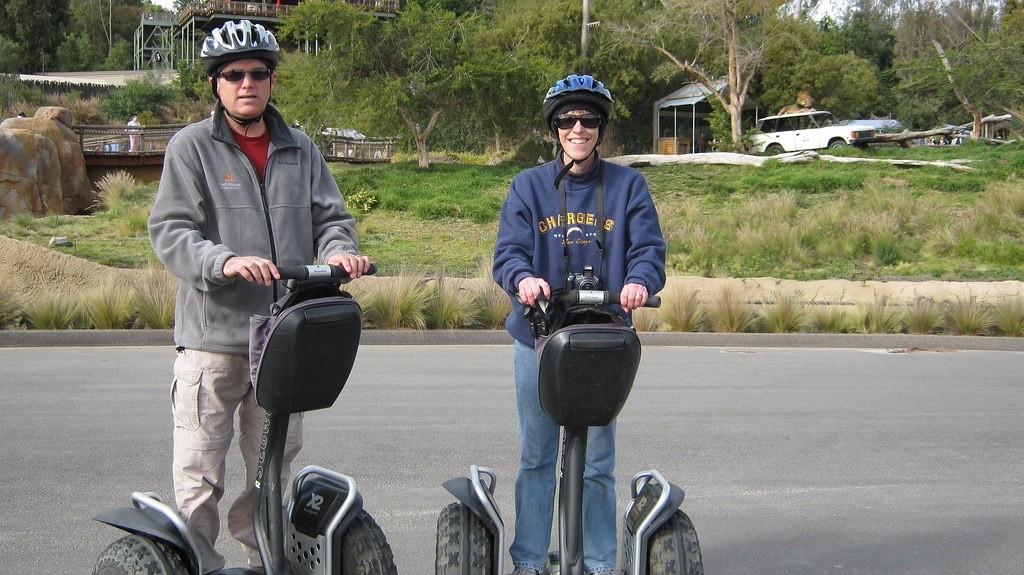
91;254;398;574
434;285;703;575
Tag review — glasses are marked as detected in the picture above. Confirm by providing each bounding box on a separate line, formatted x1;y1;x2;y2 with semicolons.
216;67;270;81
554;113;601;129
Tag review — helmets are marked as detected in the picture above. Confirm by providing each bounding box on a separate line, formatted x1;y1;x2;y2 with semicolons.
544;75;614;132
201;20;280;75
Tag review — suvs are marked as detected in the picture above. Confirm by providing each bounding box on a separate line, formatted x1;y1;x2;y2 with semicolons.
747;108;875;156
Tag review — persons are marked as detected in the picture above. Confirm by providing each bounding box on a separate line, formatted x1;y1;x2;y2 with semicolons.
145;18;370;574
125;114;141;152
492;74;668;575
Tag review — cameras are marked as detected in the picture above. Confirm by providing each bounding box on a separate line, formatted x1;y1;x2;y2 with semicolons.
566;264;601;290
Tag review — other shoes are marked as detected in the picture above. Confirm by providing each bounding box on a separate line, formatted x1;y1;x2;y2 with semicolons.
593;570;615;575
250;565;265;574
512;567;537;575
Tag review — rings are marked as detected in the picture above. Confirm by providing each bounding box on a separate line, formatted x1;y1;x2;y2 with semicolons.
355;255;360;258
635;294;642;297
